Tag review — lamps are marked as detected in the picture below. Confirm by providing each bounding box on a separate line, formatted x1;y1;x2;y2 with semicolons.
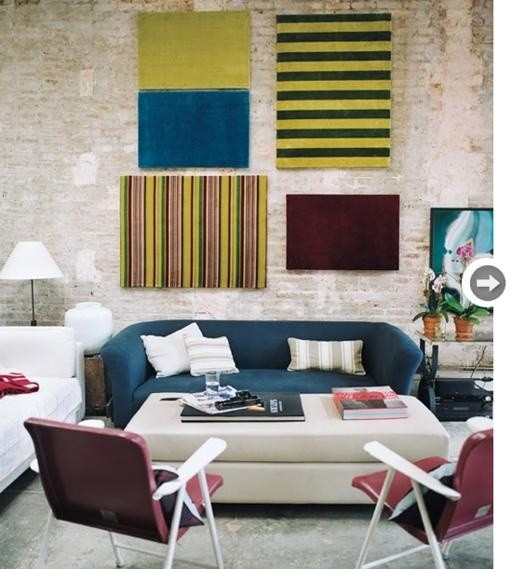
0;239;65;327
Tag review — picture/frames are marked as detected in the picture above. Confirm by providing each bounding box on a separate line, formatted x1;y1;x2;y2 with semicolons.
428;208;492;277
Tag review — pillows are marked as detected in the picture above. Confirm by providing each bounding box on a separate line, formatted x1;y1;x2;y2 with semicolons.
286;337;366;376
388;463;457;526
140;323;204;379
151;463;206;530
183;334;239;377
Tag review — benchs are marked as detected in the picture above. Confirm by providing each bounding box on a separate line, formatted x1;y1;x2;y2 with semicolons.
123;392;449;504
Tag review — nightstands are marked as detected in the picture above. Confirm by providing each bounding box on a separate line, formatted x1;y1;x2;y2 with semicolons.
85;354;111;416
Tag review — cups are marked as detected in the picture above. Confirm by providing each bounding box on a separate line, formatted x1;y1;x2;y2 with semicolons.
206;373;219;396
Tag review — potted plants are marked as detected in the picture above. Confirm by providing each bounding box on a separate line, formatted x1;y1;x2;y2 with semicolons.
413;289;448;341
444;293;489;342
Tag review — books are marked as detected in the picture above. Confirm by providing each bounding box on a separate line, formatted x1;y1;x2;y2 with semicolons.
179;391;306;422
332;385;409;419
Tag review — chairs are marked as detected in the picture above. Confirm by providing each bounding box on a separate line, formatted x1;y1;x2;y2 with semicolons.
351;427;493;569
24;418;227;569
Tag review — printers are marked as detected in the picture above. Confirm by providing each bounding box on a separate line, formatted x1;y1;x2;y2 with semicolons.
418;376;493;421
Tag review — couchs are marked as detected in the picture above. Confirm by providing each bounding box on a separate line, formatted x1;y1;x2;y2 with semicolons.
0;327;85;494
100;319;424;428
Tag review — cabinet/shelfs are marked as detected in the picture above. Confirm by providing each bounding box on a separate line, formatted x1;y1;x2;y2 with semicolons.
414;331;493;421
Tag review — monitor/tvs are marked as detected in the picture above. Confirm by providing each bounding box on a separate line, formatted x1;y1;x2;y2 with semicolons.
429;207;493;292
